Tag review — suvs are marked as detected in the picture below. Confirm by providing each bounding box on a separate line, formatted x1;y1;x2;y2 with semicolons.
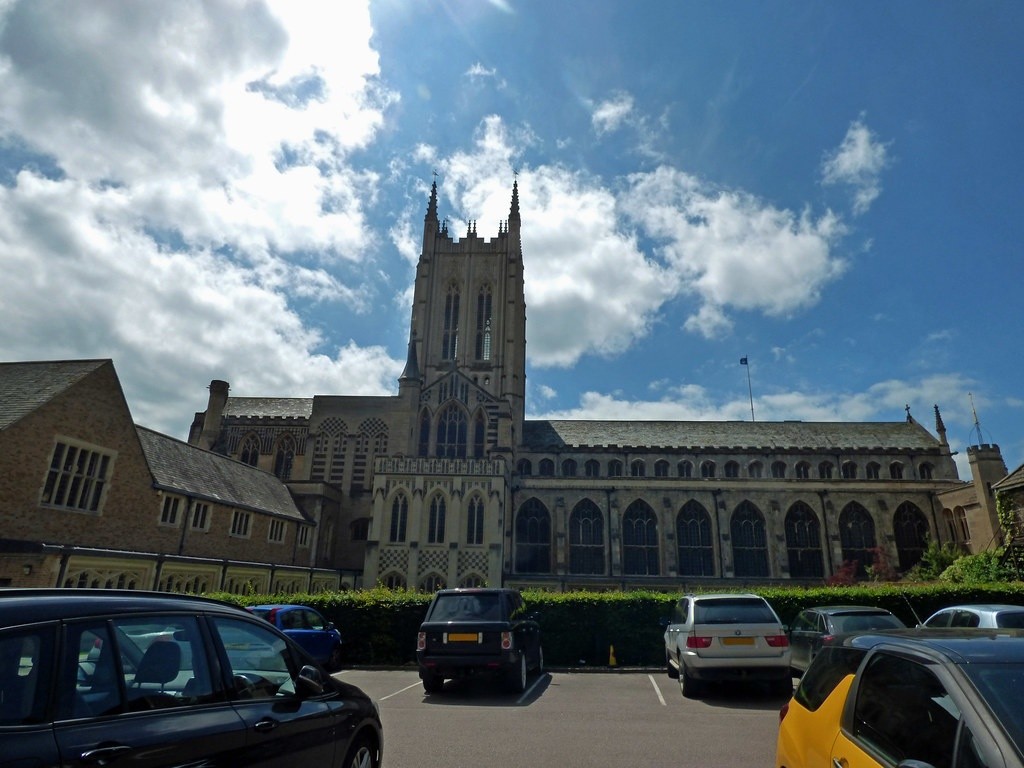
415;587;544;696
659;593;790;696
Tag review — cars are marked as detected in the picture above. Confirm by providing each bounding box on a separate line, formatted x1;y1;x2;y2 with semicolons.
0;585;383;768
89;618;189;669
784;606;905;674
776;625;1024;768
223;604;343;671
916;604;1024;627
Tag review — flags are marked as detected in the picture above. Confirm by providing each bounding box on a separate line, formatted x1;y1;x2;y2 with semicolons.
740;358;747;364
